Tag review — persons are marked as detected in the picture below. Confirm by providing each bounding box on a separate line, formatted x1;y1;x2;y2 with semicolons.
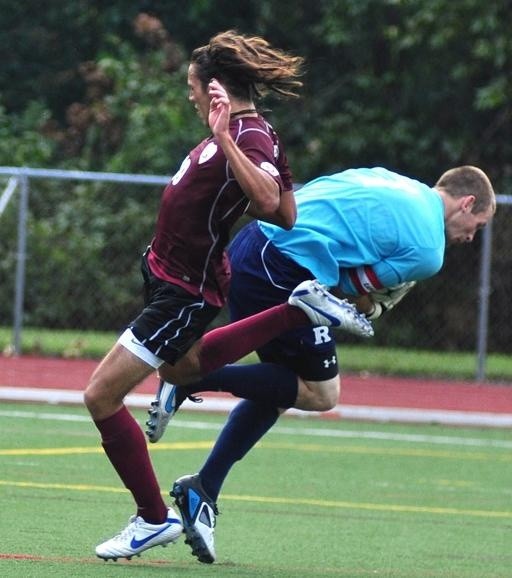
144;165;498;566
82;28;374;562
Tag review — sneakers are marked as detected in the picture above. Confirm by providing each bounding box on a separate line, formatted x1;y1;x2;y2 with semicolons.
169;474;216;563
288;278;375;340
146;378;186;444
95;502;183;561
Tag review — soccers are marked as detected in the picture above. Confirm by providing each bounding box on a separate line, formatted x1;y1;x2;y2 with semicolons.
371;279;414;302
